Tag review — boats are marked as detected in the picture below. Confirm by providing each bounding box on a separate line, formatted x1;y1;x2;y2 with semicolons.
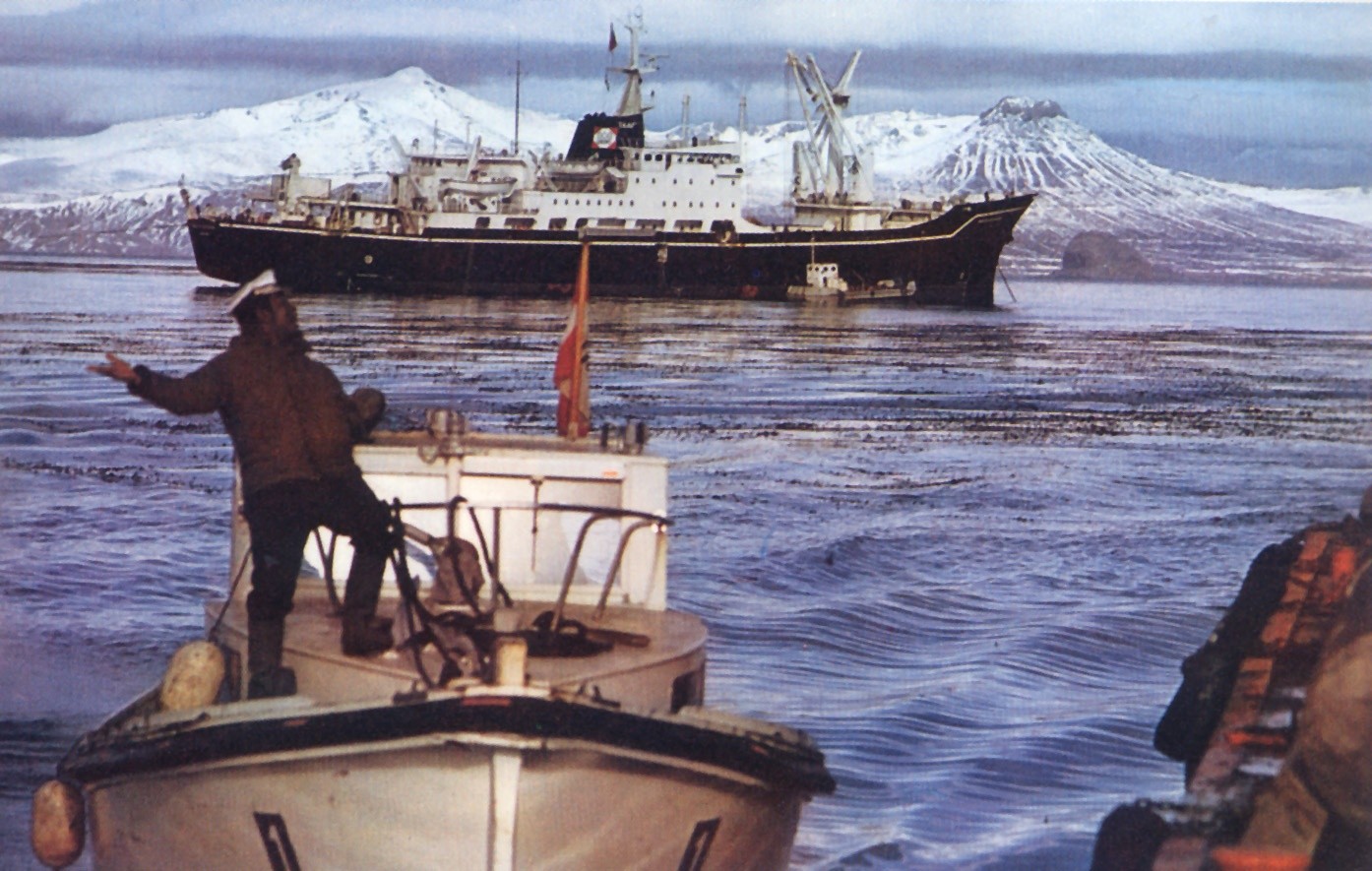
1090;482;1371;871
180;1;1041;317
25;235;846;871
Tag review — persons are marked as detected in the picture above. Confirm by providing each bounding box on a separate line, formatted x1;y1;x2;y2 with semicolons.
82;266;399;697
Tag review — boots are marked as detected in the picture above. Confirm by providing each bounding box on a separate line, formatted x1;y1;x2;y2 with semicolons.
246;619;298;700
340;579;394;657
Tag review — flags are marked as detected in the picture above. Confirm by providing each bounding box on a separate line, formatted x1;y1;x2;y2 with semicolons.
608;28;618;52
551;240;595;437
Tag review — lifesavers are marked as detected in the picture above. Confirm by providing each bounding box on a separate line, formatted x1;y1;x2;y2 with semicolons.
373;214;382;225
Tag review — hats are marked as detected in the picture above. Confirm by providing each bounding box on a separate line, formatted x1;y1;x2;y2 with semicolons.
219;267;285;324
